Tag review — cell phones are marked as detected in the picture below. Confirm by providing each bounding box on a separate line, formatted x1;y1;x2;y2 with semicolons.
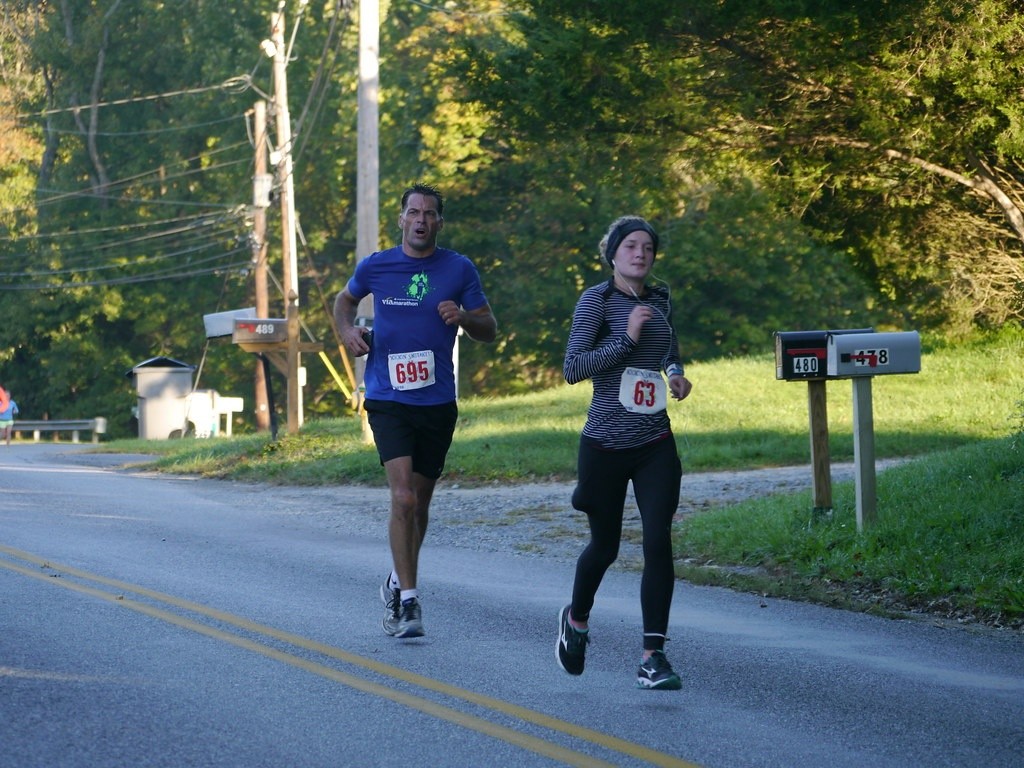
361;330;374;348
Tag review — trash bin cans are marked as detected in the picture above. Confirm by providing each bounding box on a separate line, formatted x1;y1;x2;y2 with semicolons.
126;357;197;440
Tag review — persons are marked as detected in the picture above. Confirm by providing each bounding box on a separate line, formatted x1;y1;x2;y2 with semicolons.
332;182;497;639
0;385;19;448
554;215;694;691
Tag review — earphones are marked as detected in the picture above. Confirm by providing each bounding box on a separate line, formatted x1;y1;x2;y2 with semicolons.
612;259;616;266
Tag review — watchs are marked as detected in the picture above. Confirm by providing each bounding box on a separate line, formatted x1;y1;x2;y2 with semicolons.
666;363;683;373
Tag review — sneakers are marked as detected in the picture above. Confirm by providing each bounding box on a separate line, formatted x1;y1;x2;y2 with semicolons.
635;652;682;690
379;573;401;635
554;605;590;676
395;596;424;638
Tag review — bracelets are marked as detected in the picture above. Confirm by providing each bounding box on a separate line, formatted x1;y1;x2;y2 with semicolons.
667;370;685;378
